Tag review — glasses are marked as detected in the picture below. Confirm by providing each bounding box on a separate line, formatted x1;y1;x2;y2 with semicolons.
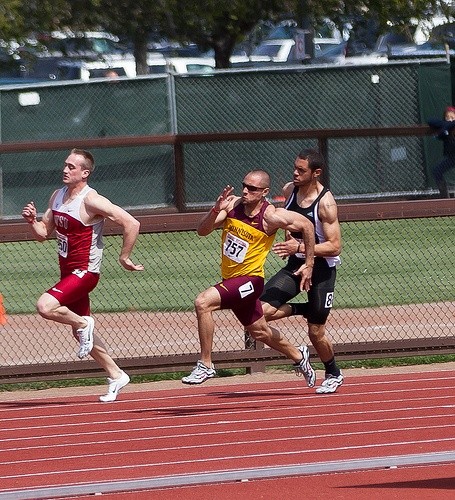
242;183;266;191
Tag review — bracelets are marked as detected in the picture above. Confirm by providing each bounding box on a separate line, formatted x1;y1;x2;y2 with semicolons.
212;207;219;213
28;218;36;224
297;243;301;252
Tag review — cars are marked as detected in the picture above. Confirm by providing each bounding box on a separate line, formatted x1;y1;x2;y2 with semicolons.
0;0;455;81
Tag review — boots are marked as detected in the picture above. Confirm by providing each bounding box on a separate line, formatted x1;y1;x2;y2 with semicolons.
431;180;450;199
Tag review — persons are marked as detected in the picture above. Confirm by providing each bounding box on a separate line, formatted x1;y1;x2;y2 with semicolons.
260;149;345;394
427;106;455;198
22;147;144;403
182;170;316;389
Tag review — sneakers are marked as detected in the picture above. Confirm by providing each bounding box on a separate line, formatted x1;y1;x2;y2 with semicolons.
293;345;316;388
315;369;343;394
182;360;216;385
100;370;130;402
76;316;94;359
